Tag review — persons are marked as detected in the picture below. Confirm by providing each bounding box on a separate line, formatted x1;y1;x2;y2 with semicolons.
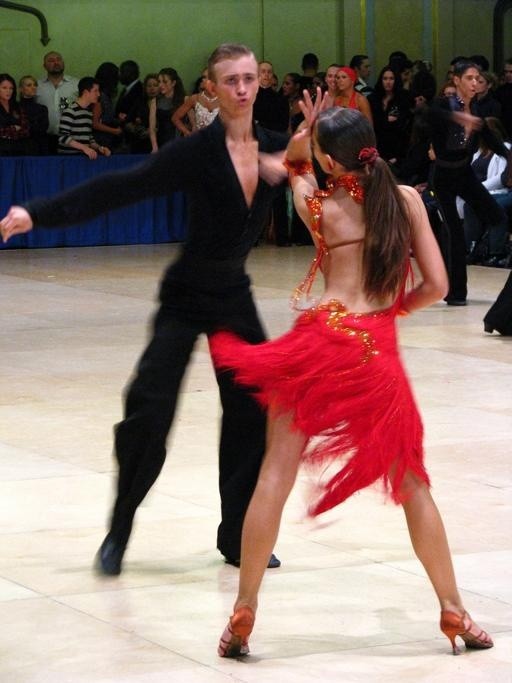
415;58;511;305
252;49;512;245
2;41;295;573
208;84;493;658
1;49;221;155
482;145;511;337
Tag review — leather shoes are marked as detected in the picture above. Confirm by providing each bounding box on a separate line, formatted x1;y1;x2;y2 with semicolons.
224;553;281;568
100;533;126;575
467;239;511;268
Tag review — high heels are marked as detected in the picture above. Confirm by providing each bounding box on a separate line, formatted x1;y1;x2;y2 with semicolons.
440;608;492;655
483;314;512;336
218;607;256;657
